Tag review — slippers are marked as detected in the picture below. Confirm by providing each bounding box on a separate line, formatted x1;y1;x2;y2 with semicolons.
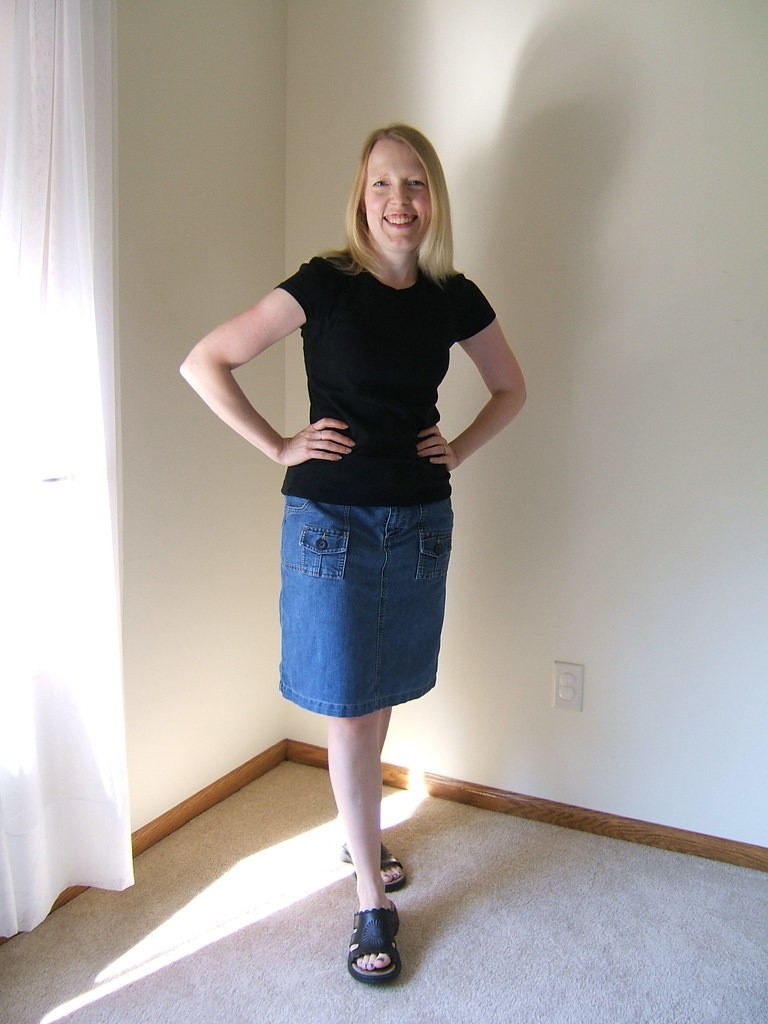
342;842;406;892
347;900;402;983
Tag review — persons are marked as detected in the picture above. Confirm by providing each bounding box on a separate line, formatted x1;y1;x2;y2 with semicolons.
179;122;531;986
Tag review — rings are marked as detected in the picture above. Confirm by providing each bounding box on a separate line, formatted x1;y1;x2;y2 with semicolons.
443;445;447;454
321;430;323;440
